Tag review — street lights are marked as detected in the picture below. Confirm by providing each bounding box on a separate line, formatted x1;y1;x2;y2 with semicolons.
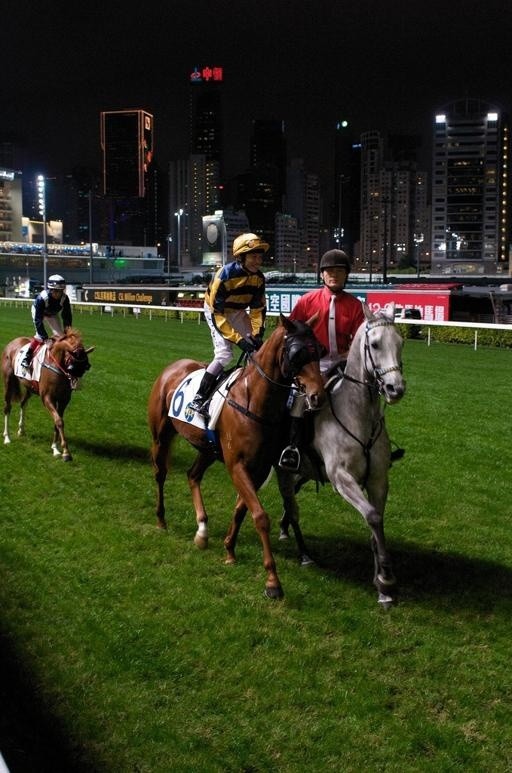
174;209;184;265
36;174;47;291
166;236;173;306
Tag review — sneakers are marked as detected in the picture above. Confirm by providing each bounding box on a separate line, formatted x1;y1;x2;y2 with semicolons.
21;357;32;368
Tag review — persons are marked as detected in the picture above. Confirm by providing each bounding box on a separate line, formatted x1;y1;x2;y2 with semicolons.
184;232;271;413
20;273;74;367
289;247;405;477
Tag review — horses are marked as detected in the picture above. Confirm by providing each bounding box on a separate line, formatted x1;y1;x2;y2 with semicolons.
259;300;406;612
0;327;96;462
147;308;330;602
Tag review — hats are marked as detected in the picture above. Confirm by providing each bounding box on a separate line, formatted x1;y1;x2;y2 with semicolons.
232;232;270;257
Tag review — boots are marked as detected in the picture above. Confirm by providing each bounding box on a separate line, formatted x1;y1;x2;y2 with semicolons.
188;371;219;421
281;413;304;467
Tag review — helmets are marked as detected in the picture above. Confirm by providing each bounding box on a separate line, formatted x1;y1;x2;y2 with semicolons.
48;274;66;290
321;248;352;271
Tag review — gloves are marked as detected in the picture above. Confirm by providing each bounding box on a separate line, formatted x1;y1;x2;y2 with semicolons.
251;335;263;351
237;338;254;352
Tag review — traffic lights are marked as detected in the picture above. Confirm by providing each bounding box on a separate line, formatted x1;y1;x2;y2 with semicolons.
114;258;127;266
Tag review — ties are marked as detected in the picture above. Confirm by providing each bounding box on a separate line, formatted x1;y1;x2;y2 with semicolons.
327;294;339;358
